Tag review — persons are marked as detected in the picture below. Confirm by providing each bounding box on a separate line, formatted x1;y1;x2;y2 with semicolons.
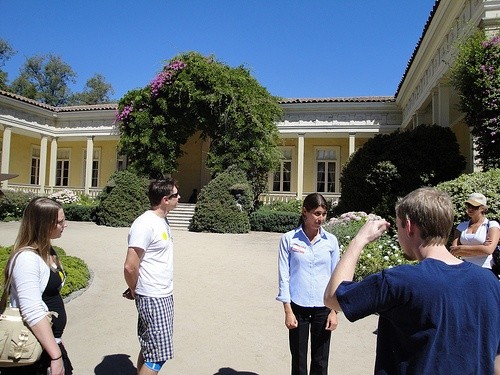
277;193;340;375
6;197;73;375
323;188;500;375
449;192;500;272
124;180;180;375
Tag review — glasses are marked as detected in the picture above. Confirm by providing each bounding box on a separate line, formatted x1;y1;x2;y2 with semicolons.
168;191;179;199
465;205;481;210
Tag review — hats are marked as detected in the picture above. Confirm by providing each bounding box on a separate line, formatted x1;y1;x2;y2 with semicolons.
464;193;487;206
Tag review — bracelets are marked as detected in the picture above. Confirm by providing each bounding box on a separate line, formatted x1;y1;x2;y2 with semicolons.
52;355;62;360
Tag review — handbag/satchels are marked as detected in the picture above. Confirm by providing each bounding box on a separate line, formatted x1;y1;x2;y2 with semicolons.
486;220;500;275
0;302;59;367
